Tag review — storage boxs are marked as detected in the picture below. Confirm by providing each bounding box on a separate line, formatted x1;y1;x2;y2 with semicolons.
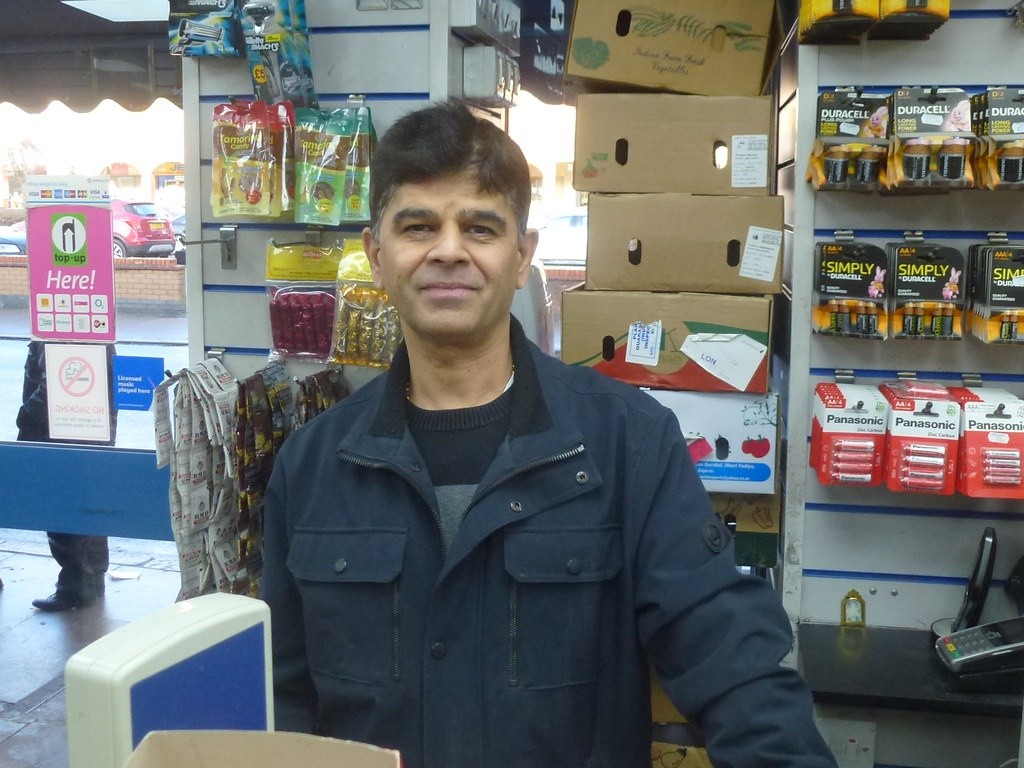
586;193;786;293
643;390;777;495
567;1;776;97
561;282;775;394
700;495;780;533
573;95;777;197
650;662;716;768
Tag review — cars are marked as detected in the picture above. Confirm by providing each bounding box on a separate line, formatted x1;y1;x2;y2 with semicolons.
0;235;28;256
164;191;186;244
533;209;587;265
10;199;175;259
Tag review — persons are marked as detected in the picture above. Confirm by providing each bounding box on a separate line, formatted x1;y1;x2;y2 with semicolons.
17;340;119;608
257;102;839;768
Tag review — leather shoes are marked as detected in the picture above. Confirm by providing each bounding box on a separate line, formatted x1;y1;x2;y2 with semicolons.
32;581;105;611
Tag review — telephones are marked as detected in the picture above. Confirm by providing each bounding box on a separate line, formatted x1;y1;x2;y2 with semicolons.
930;526;999;644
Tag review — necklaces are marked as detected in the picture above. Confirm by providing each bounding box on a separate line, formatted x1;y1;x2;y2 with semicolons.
405;378;411;398
